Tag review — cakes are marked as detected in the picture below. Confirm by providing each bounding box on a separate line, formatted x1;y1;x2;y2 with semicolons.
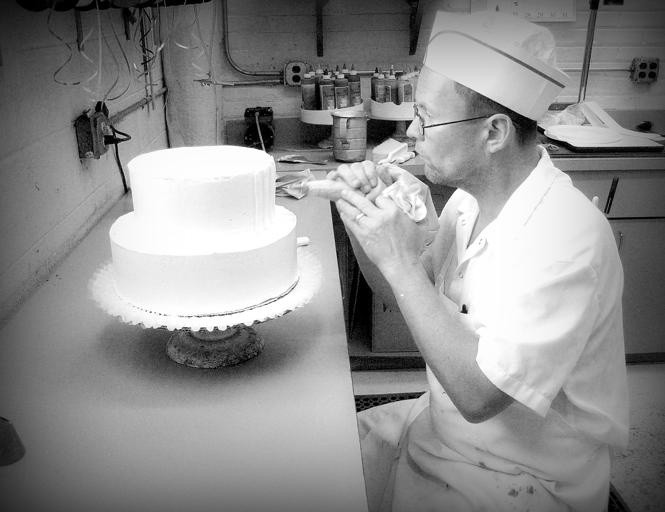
108;144;299;318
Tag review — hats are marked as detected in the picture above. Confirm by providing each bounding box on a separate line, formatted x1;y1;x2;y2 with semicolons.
423;10;572;122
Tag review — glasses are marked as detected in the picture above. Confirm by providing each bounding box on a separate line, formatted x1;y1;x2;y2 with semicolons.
412;105;521;141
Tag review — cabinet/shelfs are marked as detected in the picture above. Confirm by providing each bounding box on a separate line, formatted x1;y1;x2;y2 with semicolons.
566;170;665;364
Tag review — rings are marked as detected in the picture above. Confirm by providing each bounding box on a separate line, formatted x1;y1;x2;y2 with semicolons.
355;214;365;223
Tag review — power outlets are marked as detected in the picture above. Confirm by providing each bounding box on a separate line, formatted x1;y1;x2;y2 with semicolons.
75;113;110;159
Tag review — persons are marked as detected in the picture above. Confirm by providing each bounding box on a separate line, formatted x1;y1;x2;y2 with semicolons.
325;8;630;512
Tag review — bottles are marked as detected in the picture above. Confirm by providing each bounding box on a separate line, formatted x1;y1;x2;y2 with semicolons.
299;62;363;111
370;63;423;106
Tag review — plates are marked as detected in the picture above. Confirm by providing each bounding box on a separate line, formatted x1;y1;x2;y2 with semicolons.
543;124;623;148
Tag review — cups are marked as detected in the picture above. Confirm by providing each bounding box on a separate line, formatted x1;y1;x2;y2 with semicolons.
330;109;371;164
